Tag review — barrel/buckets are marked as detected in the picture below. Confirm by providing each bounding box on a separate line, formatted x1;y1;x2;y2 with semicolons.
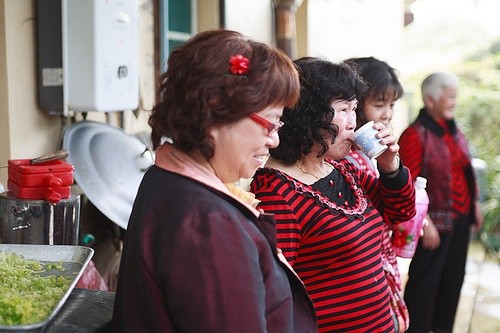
0;191;81;246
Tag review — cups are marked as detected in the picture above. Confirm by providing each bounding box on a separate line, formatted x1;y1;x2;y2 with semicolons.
349;120;388;160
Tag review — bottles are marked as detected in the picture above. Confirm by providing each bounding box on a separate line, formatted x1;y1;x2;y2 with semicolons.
390;176;430;258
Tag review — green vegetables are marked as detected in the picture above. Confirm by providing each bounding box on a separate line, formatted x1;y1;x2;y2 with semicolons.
0;251;72;326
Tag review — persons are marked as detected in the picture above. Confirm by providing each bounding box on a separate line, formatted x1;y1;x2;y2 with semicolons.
342;58;427;333
249;56;417;333
397;72;482;333
73;198;127;291
111;28;318;333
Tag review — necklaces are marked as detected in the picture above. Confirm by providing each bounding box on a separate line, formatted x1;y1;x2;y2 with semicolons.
295;161;334;181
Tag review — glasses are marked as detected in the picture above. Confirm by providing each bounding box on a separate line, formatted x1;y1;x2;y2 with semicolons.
241;108;284;137
